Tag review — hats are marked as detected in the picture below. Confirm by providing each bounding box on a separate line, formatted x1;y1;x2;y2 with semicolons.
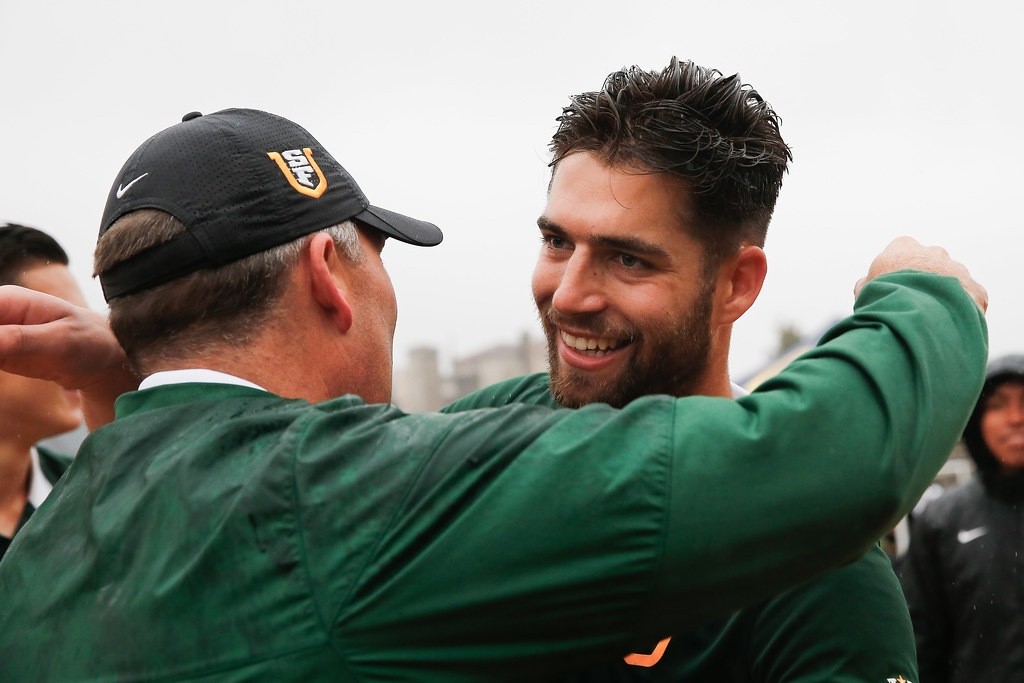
97;107;444;302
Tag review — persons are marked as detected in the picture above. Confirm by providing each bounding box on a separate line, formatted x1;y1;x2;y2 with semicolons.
890;355;1024;683
2;222;90;559
1;106;993;683
0;53;932;683
